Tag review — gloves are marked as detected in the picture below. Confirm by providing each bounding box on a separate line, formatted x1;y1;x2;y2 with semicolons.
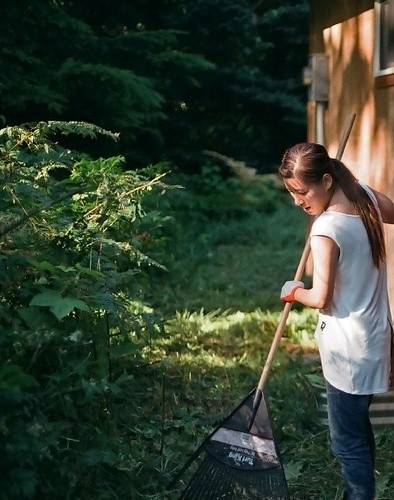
280;281;304;302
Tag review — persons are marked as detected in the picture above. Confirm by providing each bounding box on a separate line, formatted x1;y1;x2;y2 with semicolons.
276;141;394;500
